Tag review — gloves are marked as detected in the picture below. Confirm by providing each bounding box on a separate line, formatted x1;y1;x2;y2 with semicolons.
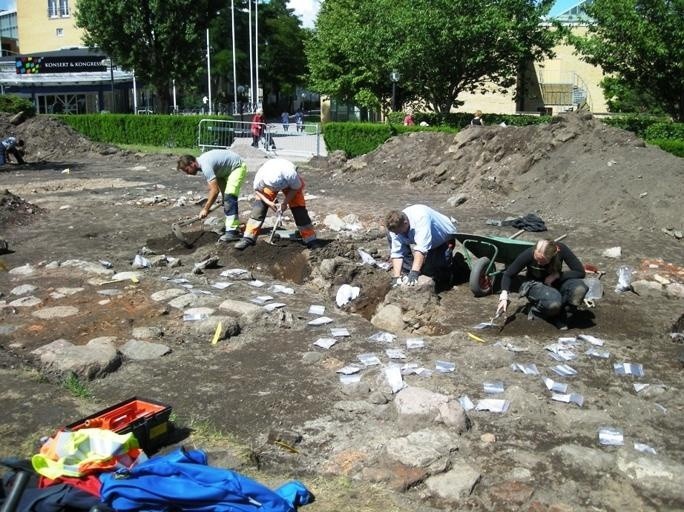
496;293;508;315
405;269;421;286
388;276;403;289
544;272;560;287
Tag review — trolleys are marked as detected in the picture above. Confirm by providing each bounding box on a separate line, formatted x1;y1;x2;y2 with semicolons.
453;225;571;298
256;125;279;159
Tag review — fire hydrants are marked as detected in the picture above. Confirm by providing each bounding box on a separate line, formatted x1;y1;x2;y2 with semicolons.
236;86;246;128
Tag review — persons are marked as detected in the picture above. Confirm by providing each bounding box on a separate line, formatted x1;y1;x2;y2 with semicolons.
495;238;589;323
234;159;316;250
176;149;247;243
470;111;483;126
251;107;267;147
403;110;414;126
384;204;472;287
294;108;304;132
0;137;26;164
280;109;290;131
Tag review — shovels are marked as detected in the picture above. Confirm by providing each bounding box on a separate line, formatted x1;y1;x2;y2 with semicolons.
578;270;606;300
472;299;511;336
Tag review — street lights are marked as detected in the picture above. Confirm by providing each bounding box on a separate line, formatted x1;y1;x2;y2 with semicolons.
390;71;401;113
100;56;117;112
171;70;179;111
301;92;306;111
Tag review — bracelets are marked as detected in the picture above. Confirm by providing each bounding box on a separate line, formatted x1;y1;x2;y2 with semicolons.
499;294;508;300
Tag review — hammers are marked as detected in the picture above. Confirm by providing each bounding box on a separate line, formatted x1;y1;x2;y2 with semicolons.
0;456;36;512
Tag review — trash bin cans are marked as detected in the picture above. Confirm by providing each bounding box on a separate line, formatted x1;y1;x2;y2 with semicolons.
537;108;552;116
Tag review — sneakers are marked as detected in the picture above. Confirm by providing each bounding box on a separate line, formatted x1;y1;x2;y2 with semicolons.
218;231;240;243
311;239;322;249
234;237;254;249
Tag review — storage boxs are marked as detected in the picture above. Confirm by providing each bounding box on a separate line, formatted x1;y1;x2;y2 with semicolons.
56;396;177;460
